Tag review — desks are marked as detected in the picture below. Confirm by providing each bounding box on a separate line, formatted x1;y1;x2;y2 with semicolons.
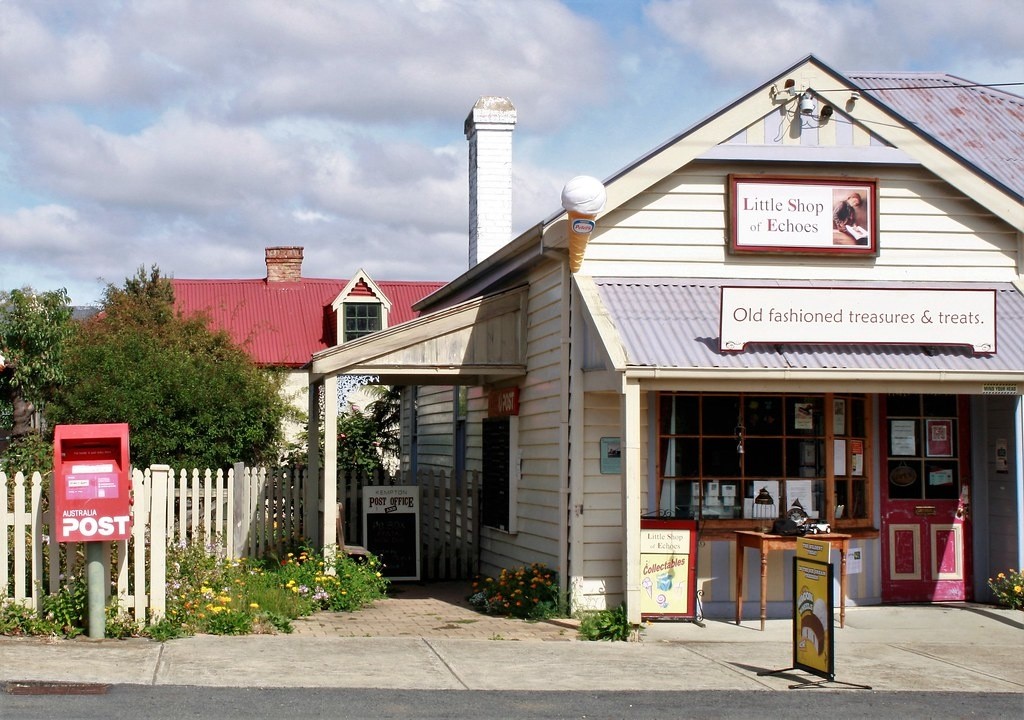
733;530;852;631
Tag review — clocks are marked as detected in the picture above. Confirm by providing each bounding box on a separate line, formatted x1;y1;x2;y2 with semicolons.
785;499;809;534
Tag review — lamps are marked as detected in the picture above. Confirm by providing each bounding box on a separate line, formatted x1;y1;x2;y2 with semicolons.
754;487;777;532
737;445;744;453
851;89;860;100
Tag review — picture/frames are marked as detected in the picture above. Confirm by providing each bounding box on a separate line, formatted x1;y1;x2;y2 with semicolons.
728;174;880;257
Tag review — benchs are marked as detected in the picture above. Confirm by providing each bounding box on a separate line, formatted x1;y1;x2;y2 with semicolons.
337;502;372;559
